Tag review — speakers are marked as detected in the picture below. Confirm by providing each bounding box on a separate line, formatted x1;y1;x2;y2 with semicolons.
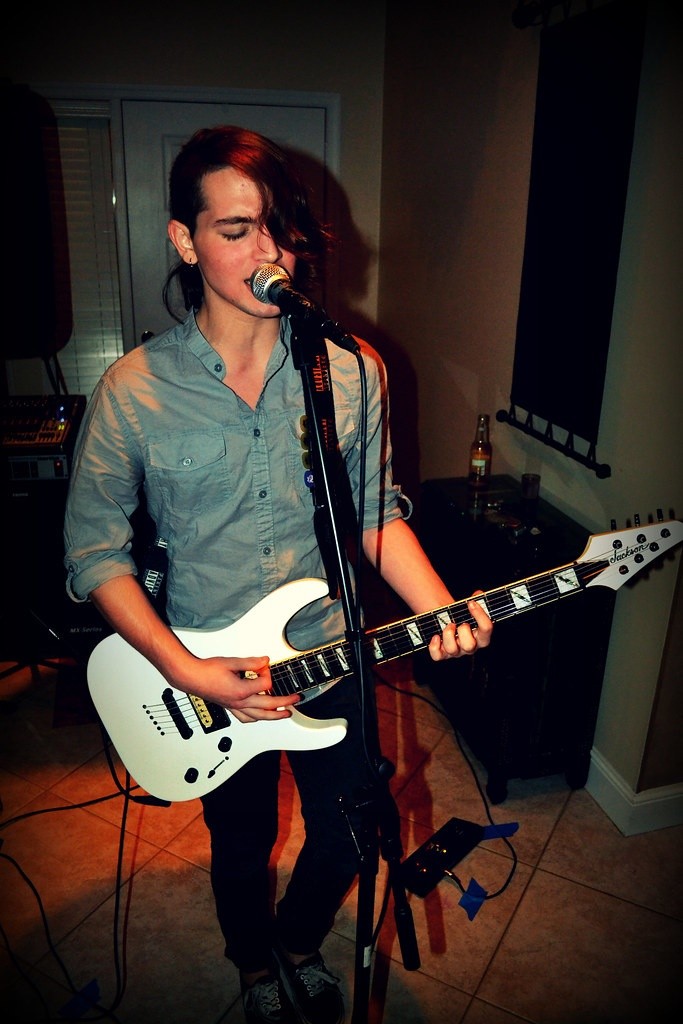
0;481;86;660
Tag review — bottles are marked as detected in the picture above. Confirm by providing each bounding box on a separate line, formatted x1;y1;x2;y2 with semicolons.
468;413;493;489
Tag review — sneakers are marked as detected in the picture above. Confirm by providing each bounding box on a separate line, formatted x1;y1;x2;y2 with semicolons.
274;946;344;1024
240;968;294;1024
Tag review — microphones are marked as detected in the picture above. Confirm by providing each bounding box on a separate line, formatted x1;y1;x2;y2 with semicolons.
250;262;361;355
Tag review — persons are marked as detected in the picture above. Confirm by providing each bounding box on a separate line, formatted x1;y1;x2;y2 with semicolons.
62;124;492;1024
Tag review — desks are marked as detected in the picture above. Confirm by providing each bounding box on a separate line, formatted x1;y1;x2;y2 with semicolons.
419;475;621;805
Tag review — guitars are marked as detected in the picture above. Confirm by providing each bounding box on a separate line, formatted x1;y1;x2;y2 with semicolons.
86;508;683;802
